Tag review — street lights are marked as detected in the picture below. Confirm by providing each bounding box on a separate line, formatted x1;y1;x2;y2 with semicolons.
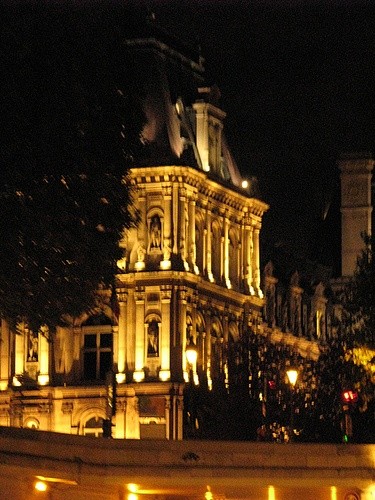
186;334;198;438
285;364;299;442
